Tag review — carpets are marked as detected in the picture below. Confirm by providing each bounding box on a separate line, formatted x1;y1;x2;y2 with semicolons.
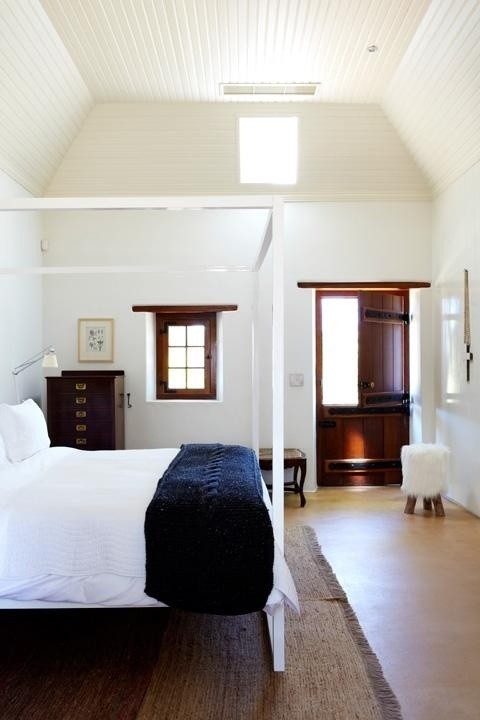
1;520;400;717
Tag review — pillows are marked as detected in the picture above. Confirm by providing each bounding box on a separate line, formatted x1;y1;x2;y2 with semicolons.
2;398;51;463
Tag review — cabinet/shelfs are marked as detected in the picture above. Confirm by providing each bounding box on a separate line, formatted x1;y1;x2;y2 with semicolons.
44;370;124;451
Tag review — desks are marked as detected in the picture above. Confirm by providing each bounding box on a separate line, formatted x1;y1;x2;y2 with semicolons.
258;449;309;507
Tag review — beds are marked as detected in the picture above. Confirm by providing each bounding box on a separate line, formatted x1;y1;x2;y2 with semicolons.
2;393;301;675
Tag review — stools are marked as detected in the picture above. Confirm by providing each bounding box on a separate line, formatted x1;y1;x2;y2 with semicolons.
397;442;453;520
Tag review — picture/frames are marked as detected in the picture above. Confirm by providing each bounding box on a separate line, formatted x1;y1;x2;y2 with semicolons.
76;318;116;364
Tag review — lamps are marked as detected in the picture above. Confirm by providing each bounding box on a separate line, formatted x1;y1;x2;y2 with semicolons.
14;344;59;382
